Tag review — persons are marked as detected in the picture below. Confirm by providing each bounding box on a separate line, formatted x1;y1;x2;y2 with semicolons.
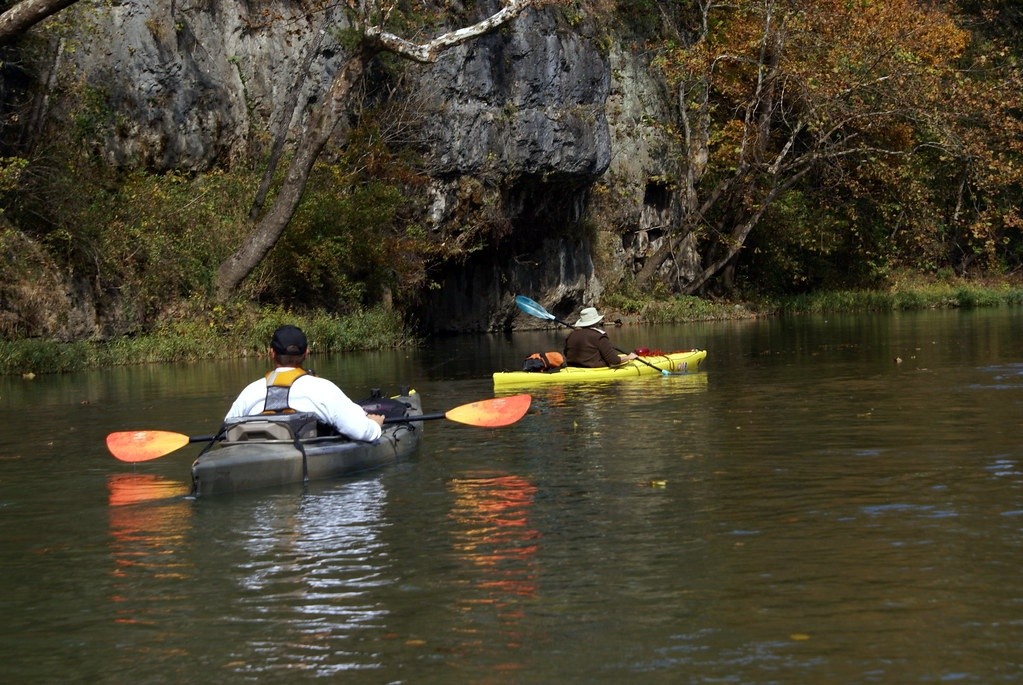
223;325;385;441
565;307;638;367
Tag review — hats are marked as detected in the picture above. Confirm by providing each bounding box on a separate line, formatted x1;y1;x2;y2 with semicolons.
270;325;307;355
571;307;604;326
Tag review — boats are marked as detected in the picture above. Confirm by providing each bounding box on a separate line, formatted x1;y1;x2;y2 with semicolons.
191;386;424;497
493;348;708;393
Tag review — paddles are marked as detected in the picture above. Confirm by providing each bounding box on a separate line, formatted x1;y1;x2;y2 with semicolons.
103;390;533;479
513;294;698;377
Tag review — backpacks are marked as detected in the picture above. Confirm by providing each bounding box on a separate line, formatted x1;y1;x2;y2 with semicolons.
522;352;567;372
